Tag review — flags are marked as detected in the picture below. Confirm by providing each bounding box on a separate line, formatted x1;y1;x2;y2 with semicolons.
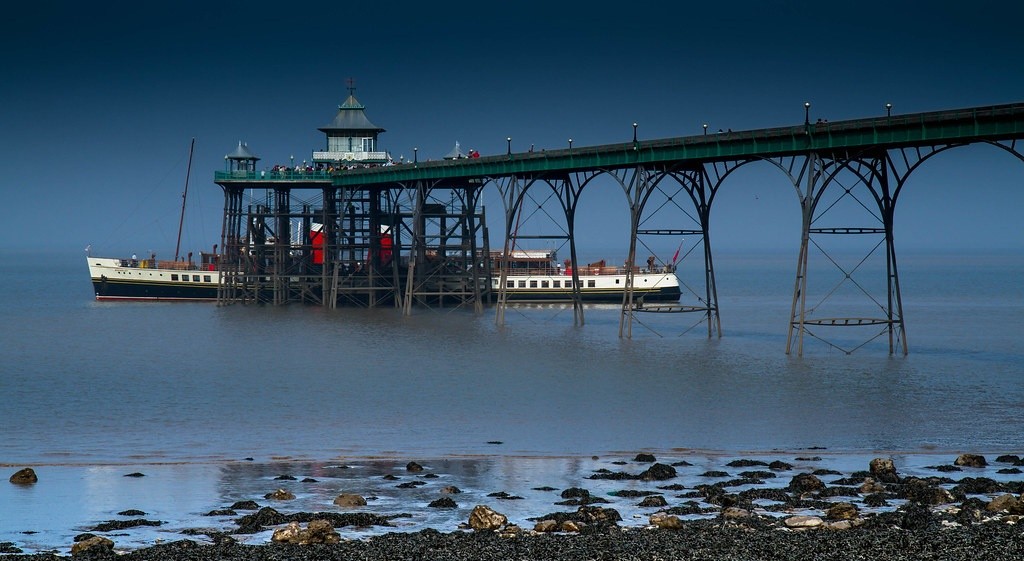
672;242;683;261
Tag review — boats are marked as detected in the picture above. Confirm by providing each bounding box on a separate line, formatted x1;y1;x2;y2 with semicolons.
85;242;685;305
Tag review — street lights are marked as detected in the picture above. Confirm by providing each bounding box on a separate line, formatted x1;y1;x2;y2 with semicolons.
225;153;228;181
633;124;639;144
290;154;294;180
507;137;512;156
885;103;891;127
804;103;812;130
568;138;572;148
415;147;417;163
704;124;707;134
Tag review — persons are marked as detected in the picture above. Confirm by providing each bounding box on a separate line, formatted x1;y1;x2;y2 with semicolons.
467;149;480;158
131;253;138;267
260;164;395;179
